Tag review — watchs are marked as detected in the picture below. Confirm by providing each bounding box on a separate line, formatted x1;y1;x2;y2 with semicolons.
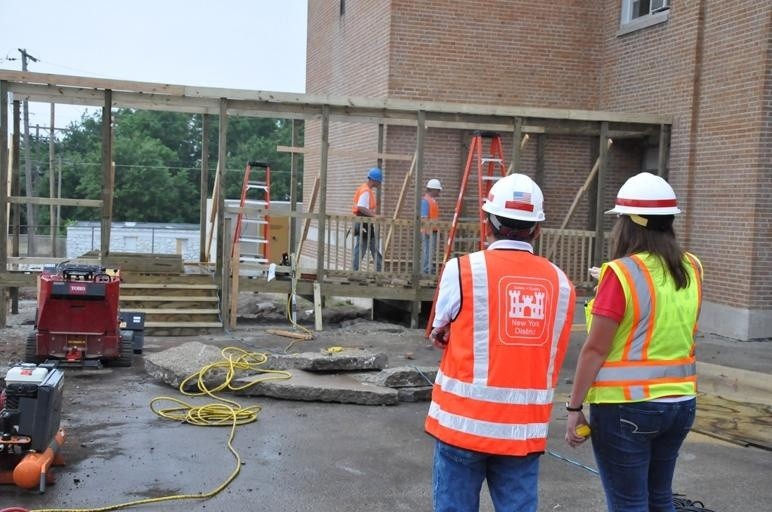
566;401;583;411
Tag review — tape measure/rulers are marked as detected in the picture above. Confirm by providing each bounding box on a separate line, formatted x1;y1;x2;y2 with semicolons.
574;424;591;437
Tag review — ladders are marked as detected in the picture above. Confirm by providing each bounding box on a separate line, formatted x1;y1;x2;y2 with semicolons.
229;160;272;277
424;130;507;340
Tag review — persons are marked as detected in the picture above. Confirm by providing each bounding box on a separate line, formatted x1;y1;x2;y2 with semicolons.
352;168;383;272
421;179;442;275
565;173;703;512
424;174;575;511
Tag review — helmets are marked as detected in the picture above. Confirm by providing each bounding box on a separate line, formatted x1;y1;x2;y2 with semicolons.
426;179;443;189
482;174;545;222
368;168;383;182
604;172;682;215
